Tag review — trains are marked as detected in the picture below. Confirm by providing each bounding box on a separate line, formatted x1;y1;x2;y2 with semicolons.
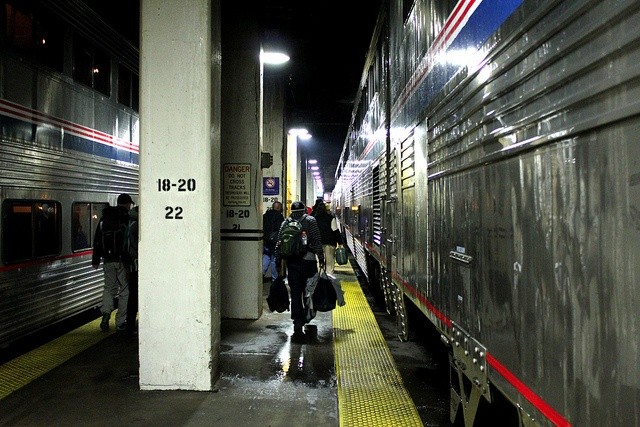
329;0;639;427
1;0;141;349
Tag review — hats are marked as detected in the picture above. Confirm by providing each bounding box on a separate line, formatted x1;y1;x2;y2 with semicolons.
117;194;135;204
291;202;306;212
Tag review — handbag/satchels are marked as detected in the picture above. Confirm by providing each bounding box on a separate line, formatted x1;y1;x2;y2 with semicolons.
265;276;290;313
313;267;338;312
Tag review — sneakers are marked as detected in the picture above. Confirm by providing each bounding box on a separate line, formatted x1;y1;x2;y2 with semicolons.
99;314;111;330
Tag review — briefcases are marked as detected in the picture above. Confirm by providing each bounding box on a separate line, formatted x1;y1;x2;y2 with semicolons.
336;244;347;265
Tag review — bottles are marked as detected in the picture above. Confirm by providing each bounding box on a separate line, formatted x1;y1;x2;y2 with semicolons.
301;232;308;245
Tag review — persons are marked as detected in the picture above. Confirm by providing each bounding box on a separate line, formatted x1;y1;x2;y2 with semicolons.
314;201;343;279
91;193;135;332
262;201;285;282
124;204;138;337
276;200;325;332
310;199;323;216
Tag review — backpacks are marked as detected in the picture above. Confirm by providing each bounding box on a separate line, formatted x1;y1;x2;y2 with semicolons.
279;214;309;260
